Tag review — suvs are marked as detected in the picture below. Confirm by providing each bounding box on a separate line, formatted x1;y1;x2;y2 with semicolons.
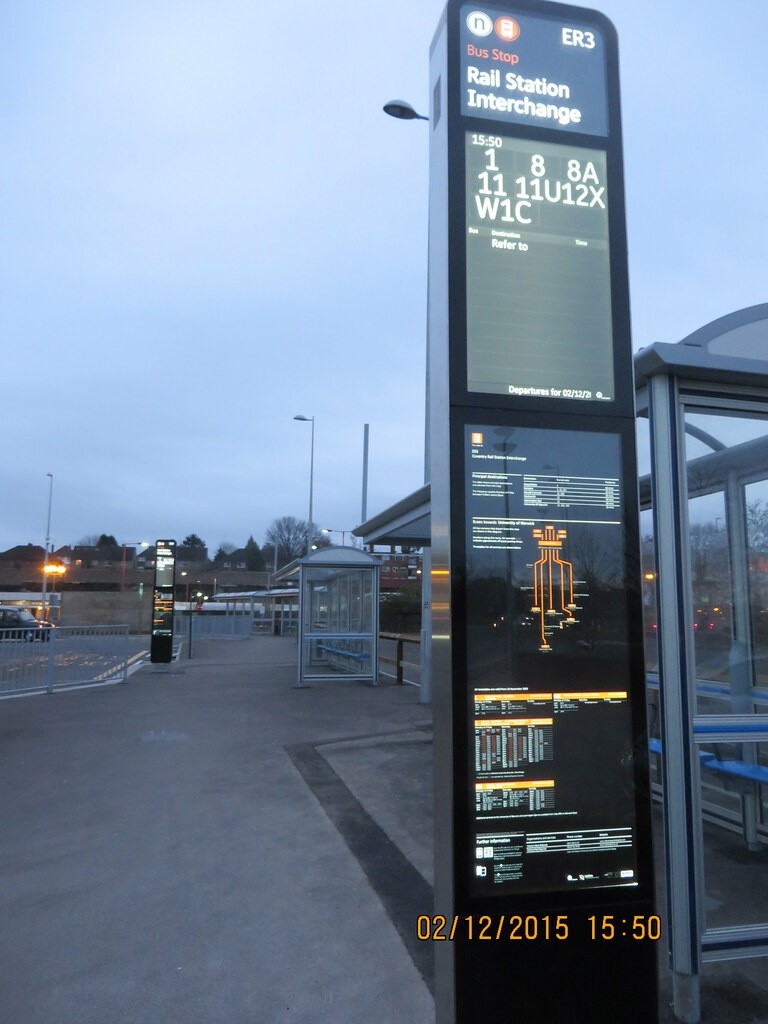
0;604;55;642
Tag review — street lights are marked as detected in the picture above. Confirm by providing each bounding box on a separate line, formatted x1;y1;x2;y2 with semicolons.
181;572;193;658
322;529;353;546
294;414;315;556
38;472;54;639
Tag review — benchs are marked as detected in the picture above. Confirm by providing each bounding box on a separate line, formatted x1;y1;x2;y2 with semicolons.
648;738;768;854
317;644;371;675
